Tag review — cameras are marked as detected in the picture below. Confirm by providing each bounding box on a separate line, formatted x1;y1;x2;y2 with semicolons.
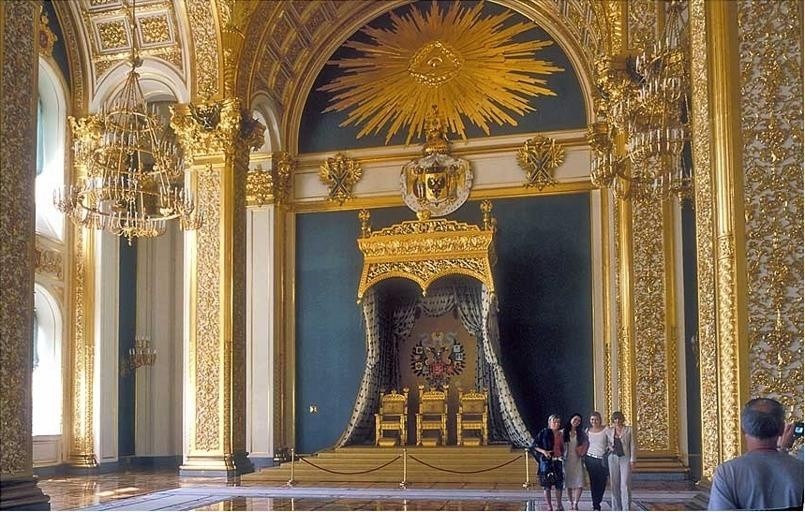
794;422;804;436
540;452;564;462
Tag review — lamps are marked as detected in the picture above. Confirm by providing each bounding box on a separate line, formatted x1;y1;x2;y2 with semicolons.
129;334;156;371
53;0;204;247
590;0;695;206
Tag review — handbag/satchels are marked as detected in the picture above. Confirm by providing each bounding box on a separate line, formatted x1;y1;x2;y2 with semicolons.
601;449;611;467
539;453;558;485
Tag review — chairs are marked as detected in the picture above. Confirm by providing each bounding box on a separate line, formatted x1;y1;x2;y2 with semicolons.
455;387;488;447
374;387;410;447
415;384;449;447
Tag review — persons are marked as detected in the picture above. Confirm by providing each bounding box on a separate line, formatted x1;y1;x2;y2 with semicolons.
706;395;804;511
604;411;638;510
777;421;804;462
558;412;590;510
529;414;565;510
583;411;609;510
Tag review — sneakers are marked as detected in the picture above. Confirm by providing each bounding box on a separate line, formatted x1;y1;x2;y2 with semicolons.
547;501;600;510
